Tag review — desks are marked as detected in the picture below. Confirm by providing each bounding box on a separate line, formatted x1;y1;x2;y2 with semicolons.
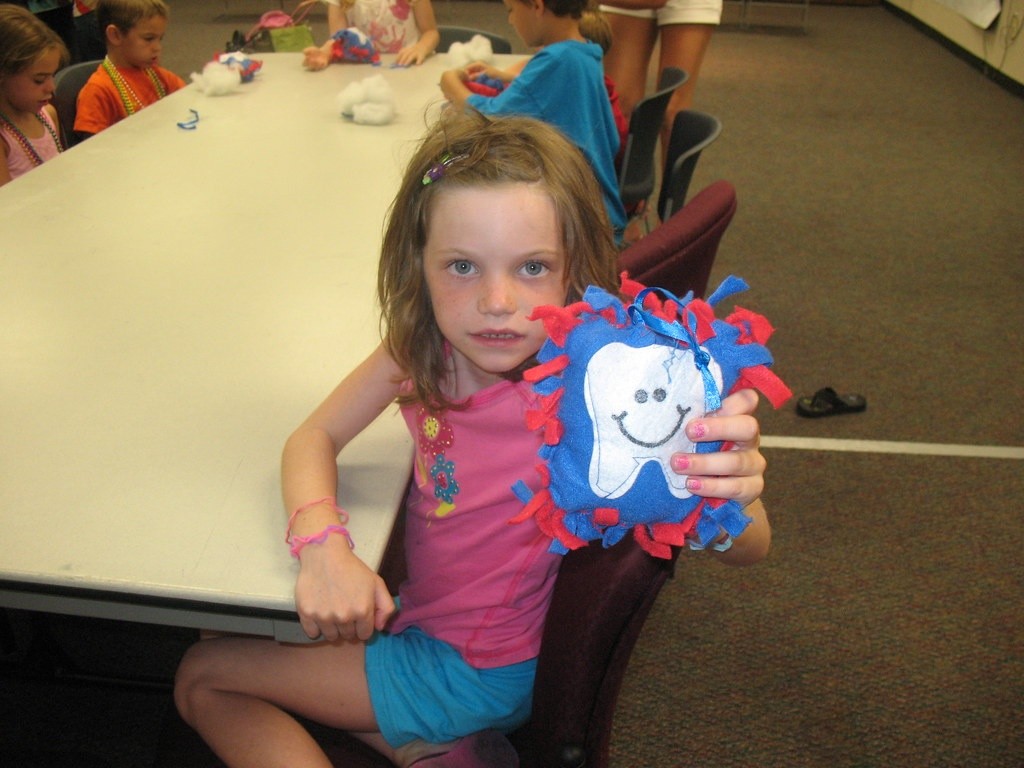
0;54;531;643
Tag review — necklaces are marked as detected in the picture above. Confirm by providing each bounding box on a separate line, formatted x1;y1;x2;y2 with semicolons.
0;110;64;167
103;55;167;116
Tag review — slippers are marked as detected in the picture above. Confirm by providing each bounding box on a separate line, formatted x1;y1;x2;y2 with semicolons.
797;387;866;417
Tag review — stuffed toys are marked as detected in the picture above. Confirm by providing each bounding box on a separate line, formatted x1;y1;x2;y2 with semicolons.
330;27;380;66
205;50;262;83
464;68;511;97
524;270;793;552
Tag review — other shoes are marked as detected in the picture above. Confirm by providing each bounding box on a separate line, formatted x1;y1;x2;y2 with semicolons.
406;730;520;768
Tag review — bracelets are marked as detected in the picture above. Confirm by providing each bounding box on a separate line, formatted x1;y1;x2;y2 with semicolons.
285;498;355;557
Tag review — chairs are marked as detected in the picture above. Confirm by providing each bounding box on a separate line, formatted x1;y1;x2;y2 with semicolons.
435;25;515;56
51;57;103;149
611;181;736;300
658;109;721;227
614;67;690;235
510;529;684;767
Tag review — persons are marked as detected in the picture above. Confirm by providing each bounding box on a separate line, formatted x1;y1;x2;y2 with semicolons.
505;12;653;221
439;0;629;250
593;0;724;245
73;0;187;140
301;0;440;72
173;103;774;768
0;4;72;186
